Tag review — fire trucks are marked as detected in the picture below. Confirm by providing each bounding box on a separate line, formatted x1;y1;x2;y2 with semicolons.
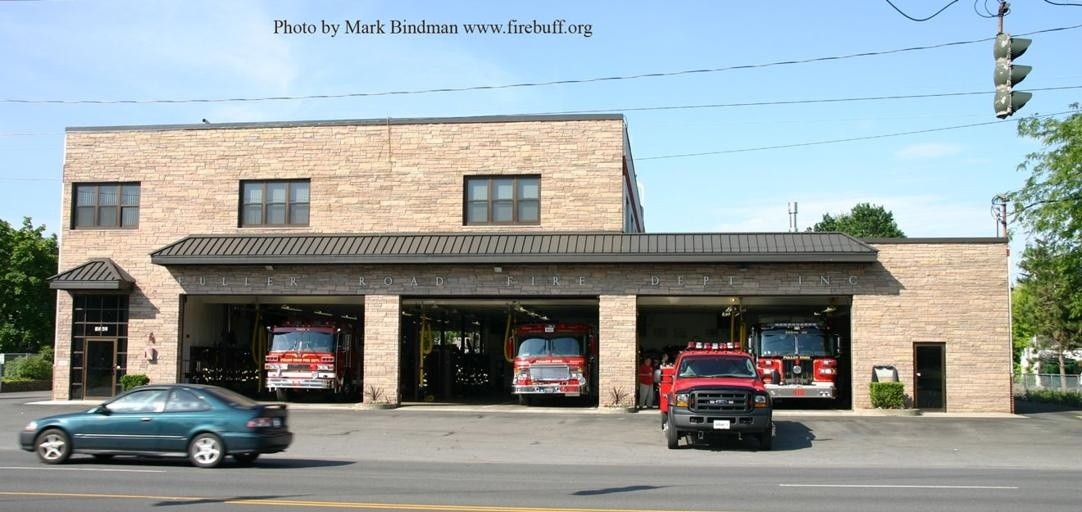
749;322;842;408
263;321;356;399
506;322;596;404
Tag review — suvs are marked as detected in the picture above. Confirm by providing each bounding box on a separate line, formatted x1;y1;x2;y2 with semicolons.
667;341;773;450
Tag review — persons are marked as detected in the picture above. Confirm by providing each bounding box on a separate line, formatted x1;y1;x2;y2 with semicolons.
637;356;655;409
658;352;668;369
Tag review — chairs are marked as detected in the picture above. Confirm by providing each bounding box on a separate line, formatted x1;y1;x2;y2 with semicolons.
685;366;705;375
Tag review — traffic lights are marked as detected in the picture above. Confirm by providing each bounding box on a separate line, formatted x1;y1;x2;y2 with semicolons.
994;34;1032;119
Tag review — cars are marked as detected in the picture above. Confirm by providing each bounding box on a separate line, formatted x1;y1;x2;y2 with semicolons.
19;385;292;469
